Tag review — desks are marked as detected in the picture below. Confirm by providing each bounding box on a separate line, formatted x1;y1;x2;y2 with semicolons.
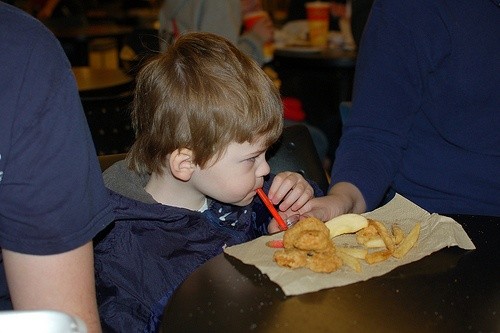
72;66;132;117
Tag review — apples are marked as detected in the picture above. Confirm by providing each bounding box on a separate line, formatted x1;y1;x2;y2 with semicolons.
323;213;368;238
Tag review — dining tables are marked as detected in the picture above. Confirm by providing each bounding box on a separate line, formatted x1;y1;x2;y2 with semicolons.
242;21;354;68
158;213;500;333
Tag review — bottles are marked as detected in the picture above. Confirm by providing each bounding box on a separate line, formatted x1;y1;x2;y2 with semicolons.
278;215;298;231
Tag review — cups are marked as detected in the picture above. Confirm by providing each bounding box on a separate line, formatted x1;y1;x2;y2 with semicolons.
243;11;273;62
306;3;329;48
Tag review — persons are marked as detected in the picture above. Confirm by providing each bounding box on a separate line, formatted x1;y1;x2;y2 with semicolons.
267;0;500;235
0;5;117;332
95;29;325;333
158;0;272;69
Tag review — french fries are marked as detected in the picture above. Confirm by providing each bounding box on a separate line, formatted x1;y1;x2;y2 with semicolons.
337;219;419;272
